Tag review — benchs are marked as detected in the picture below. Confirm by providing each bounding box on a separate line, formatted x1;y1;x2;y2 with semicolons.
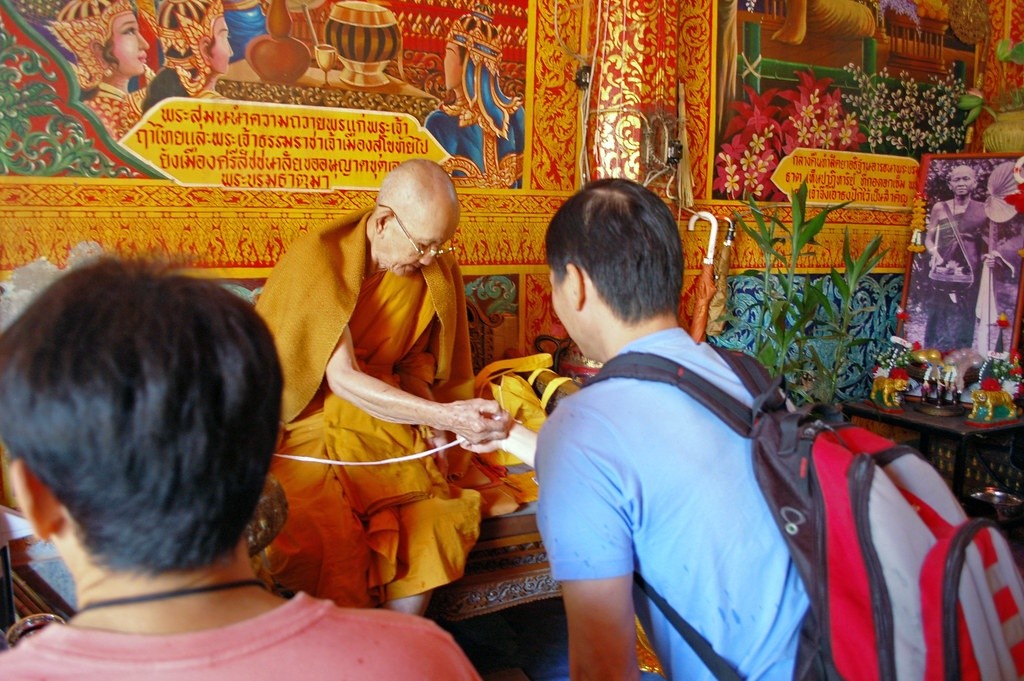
0;296;581;626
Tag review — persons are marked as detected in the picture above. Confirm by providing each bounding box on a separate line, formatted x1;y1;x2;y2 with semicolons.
253;158;513;617
924;165;989;354
0;258;481;681
456;178;810;681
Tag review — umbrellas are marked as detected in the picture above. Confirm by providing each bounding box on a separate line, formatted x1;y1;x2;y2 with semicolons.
685;210;737;345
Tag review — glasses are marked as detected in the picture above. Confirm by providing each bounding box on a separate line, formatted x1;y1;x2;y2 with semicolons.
379;202;456;258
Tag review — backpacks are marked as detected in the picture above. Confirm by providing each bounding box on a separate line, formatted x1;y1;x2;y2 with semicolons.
581;344;1024;681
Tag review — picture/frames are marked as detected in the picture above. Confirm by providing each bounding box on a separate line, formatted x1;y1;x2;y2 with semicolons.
895;152;1024;409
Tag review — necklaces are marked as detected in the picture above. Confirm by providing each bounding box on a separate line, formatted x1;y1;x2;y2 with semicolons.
80;579;266;613
